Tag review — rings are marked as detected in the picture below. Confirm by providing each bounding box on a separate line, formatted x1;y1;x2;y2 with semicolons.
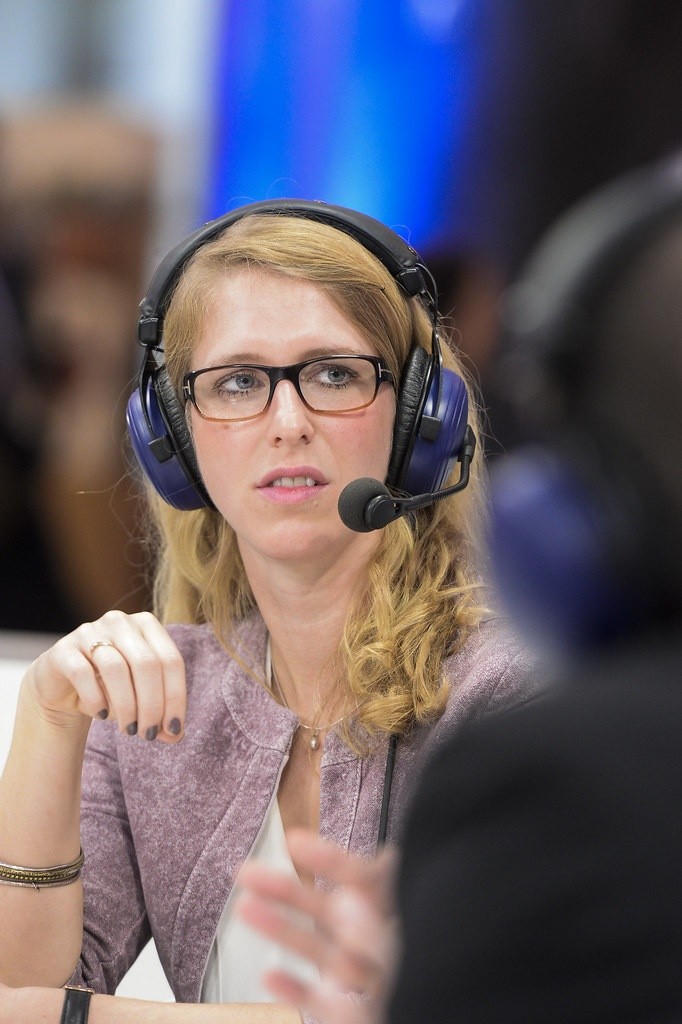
89;640;113;657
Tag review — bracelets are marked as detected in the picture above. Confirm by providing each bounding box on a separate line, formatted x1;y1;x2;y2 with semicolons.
61;984;95;1024
0;846;84;893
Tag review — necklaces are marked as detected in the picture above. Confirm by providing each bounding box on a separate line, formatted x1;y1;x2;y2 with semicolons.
271;660;371;750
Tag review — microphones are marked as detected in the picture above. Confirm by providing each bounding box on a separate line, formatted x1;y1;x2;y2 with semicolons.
338;456;473;533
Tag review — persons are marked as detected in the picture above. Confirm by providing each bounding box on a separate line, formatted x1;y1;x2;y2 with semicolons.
232;160;682;1024
0;200;501;1024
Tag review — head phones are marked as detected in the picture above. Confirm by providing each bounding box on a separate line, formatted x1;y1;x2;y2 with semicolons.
125;200;477;511
480;161;682;673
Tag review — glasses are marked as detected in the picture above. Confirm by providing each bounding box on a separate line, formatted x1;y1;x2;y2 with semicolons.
183;355;396;422
